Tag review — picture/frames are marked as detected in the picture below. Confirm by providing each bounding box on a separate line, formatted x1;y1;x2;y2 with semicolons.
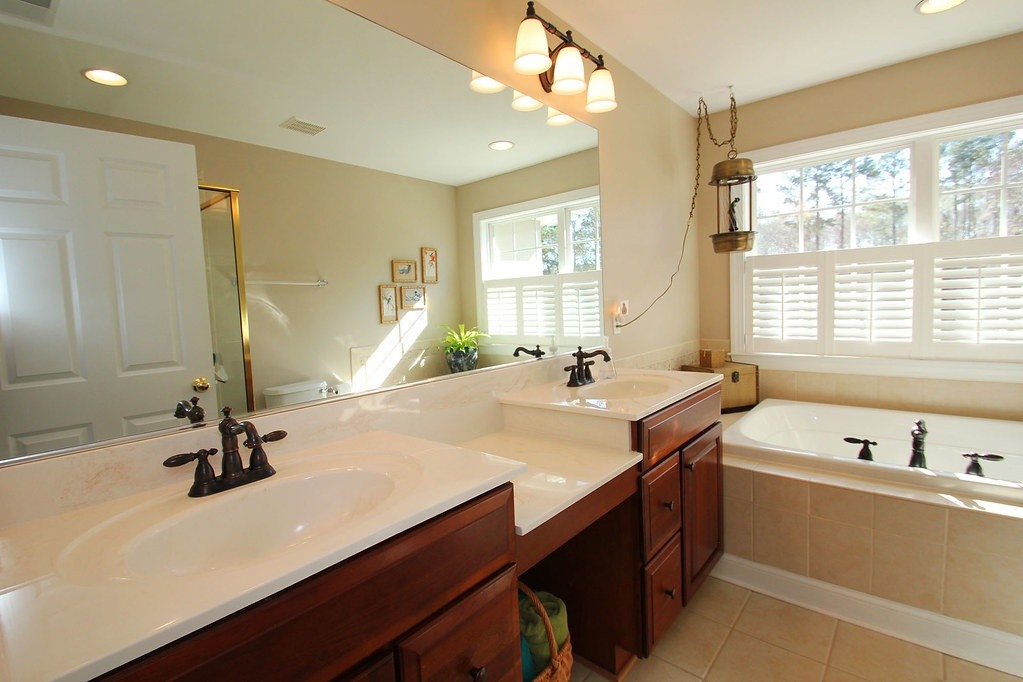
392;260;418;282
379;285;399;324
421;248;438;284
401;286;426;310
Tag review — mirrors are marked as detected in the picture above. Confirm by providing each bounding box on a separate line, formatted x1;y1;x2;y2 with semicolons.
0;0;607;467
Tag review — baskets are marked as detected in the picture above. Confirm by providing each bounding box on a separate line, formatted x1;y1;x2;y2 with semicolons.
517;582;573;682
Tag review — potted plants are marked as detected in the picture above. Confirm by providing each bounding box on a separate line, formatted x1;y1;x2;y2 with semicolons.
436;323;492;373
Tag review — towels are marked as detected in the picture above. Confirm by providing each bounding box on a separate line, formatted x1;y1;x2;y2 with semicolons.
519;592;568;682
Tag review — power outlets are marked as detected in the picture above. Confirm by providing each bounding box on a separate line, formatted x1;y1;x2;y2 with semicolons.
620;300;629;314
613;312;621;334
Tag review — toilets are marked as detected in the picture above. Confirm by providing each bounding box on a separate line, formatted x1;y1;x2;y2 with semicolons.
260;379;338;410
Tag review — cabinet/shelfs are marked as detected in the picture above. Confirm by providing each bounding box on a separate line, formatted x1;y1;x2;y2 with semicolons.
88;377;724;682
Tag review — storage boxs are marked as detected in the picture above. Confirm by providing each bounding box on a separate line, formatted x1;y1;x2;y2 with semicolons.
700;349;724;367
681;362;759;413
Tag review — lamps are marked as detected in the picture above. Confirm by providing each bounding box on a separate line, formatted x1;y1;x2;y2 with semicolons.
585;54;617;113
514;1;552;77
551;30;587;95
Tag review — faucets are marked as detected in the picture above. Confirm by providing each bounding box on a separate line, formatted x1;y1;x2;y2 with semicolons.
563;346;612;387
175;395;207;425
513;345;545;359
910;420;928;471
163;405;287;498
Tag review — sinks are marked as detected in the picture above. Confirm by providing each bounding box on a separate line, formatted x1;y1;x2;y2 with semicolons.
577;379;675;399
57;465;401;596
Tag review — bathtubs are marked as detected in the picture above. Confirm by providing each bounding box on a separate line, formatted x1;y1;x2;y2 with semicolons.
721;395;1023;518
